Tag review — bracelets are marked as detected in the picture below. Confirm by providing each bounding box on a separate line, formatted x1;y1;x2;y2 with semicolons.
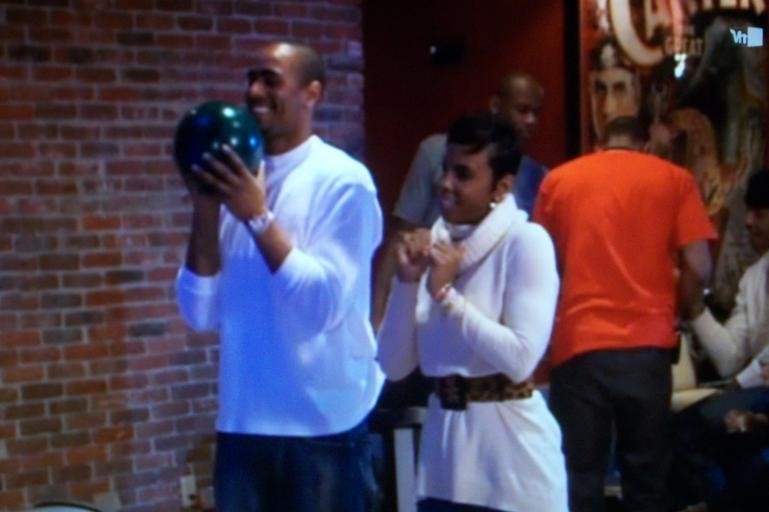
435;281;453;302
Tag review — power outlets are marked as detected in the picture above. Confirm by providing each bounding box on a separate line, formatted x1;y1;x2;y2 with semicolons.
180;475;199;508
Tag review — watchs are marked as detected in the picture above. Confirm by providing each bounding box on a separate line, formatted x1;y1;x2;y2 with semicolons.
245;211;275;235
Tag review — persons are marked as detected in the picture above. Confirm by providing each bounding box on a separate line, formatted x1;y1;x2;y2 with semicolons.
390;70;551;231
375;109;570;512
672;168;769;511
531;113;719;511
175;40;388;512
587;36;643;141
